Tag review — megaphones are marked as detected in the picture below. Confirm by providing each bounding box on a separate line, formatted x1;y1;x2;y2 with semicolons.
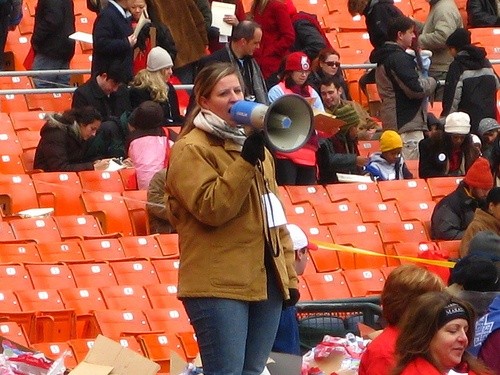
230;93;314;153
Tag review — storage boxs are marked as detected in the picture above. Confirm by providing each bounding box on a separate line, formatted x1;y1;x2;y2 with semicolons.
169;350;275;375
68;334;160;375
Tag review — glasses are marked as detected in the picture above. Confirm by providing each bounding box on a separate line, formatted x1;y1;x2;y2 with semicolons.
325;61;341;68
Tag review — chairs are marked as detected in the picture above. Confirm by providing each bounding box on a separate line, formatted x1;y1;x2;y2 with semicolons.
0;0;500;375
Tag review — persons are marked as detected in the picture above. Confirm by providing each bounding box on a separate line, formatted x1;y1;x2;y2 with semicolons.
431;157;493;240
233;0;381;139
459;187;500;259
270;223;308;356
447;229;500;375
418;112;500;187
34;101;164;175
390;291;499;375
347;0;500;160
0;0;24;66
71;45;186;127
87;0;239;96
362;130;414;181
198;20;268;106
439;27;500;134
267;53;341;186
315;105;370;186
145;60;300;375
30;0;75;88
358;263;447;375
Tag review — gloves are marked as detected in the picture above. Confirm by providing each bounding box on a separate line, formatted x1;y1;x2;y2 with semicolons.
241;129;267;163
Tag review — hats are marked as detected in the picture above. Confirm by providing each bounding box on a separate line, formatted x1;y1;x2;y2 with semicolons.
285;52;311;72
444;111;471;134
467;229;500;262
478;118;500;137
284;223;308;251
446;27;472;50
334;102;360;138
146;46;174;72
131;101;165;129
379;130;402;152
451;254;498;291
463;157;493;188
